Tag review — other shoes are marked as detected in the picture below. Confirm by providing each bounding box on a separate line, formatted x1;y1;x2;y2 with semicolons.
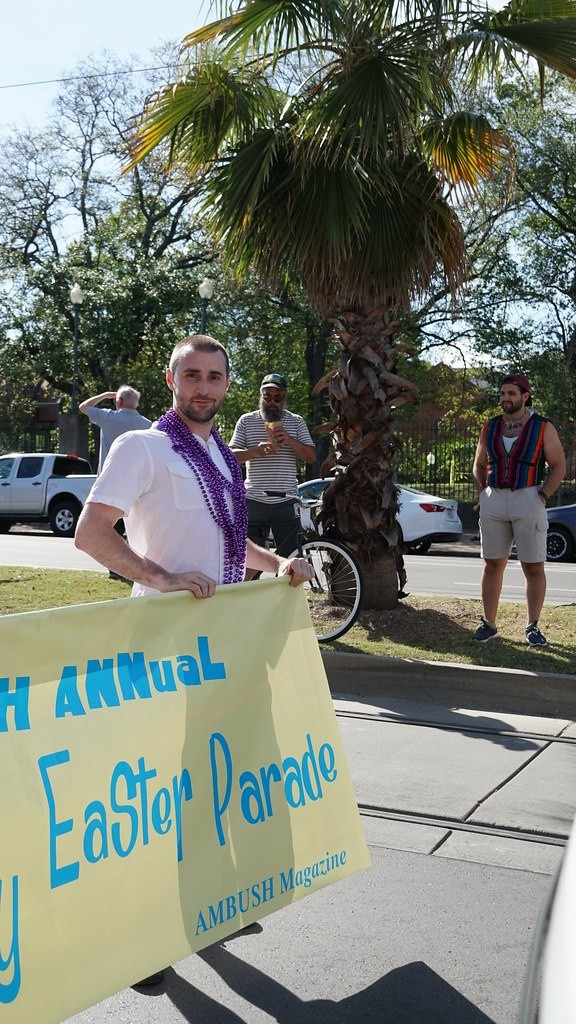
140;971;164;987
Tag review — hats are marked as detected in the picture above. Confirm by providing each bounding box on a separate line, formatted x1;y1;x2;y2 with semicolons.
501;375;532;406
261;374;286;390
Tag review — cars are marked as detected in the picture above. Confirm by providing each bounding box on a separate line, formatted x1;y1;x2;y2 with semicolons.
296;477;462;556
544;503;576;563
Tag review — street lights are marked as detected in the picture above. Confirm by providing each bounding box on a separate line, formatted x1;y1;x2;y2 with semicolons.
69;280;84;411
197;278;213;335
426;451;435;492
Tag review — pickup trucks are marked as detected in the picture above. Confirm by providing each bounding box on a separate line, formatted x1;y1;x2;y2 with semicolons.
0;453;98;538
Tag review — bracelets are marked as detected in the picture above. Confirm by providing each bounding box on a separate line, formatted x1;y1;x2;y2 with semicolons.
538;491;548;501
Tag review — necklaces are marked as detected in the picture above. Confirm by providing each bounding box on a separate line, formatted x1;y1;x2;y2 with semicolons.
499;408;527;430
157;409;249;585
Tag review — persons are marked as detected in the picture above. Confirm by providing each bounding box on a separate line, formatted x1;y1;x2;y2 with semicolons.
472;375;567;646
229;374;316;580
79;385;153;578
74;335;315;986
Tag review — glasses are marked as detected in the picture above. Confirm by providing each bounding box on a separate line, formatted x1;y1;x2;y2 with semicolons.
263;396;284;403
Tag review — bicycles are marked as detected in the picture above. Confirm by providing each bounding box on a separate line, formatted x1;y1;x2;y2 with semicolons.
248;490;364;644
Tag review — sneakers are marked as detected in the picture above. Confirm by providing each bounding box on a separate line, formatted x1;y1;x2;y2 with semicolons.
524;619;547;646
471;618;498;643
